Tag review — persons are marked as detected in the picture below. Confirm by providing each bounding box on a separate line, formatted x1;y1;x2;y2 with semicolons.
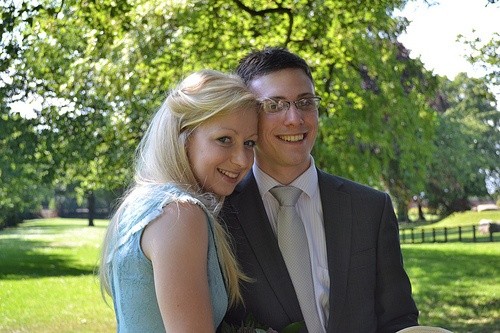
218;49;419;333
98;68;259;333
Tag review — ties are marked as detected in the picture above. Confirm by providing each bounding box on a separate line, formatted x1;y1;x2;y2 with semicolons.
269;186;324;333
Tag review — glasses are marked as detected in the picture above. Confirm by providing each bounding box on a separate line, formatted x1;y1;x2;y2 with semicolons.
257;95;321;114
